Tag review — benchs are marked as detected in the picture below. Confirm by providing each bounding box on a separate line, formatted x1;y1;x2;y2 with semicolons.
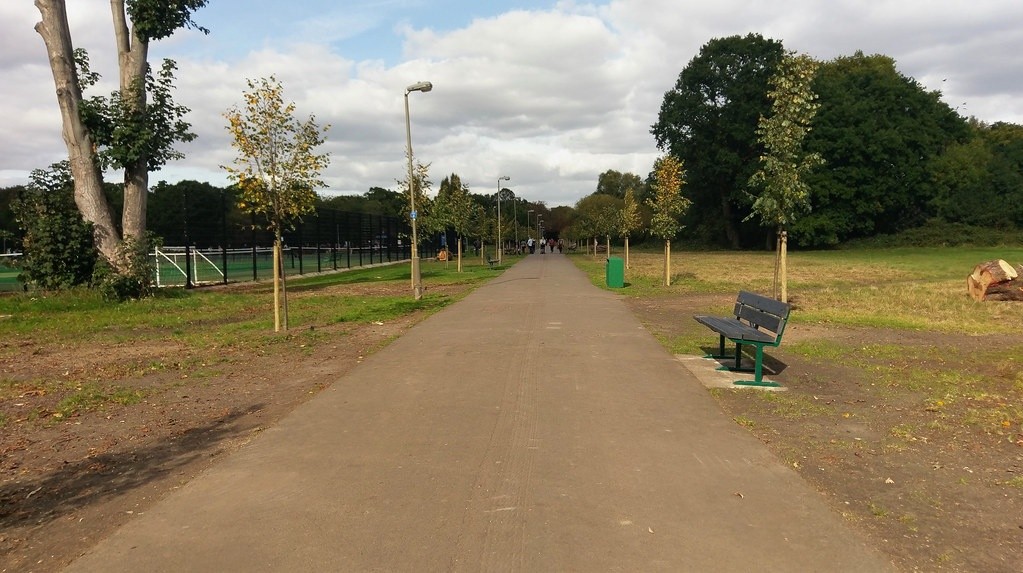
485;253;500;266
692;289;791;387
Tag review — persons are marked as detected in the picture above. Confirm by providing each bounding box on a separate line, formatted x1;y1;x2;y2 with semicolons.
473;238;481;256
558;238;564;253
527;237;534;254
549;238;555;252
540;237;547;254
566;242;577;255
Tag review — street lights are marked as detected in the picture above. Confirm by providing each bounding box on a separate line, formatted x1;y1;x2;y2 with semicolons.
514;196;521;255
403;80;433;301
497;176;510;265
528;209;535;235
537;214;545;249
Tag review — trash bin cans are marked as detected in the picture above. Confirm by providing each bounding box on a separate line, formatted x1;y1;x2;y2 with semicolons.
605;257;624;287
